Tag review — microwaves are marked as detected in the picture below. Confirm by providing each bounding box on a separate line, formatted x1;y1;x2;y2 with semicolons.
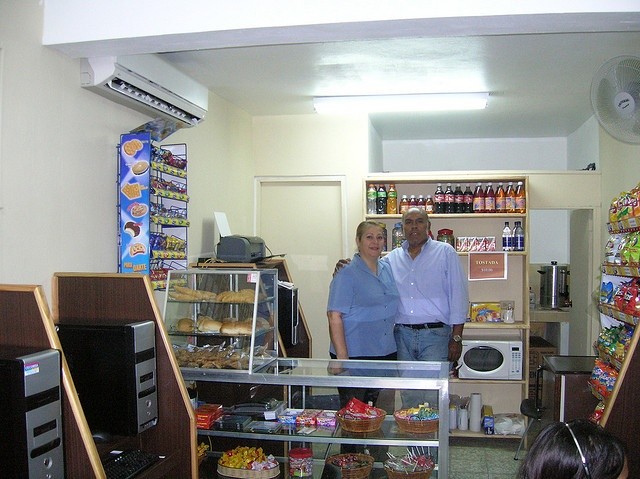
458;334;523;380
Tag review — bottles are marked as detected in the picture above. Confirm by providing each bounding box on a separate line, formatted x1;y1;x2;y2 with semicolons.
392;223;403;249
502;221;525;251
377;223;387;251
367;182;524;213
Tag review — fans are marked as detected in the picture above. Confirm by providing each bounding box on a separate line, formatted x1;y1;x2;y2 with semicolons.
591;54;640;146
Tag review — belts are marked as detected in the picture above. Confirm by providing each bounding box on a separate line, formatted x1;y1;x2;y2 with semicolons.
401;322;445;330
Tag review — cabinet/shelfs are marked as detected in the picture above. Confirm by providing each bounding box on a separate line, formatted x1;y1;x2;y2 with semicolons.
119;134;189;292
162;267;278;374
183;359;449;479
361;172;531;440
592;218;640;427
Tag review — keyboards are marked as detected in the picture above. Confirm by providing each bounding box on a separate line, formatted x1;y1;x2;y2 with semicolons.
100;449;164;477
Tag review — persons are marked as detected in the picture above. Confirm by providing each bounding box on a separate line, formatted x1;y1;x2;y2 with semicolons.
325;220;400;479
331;210;470;463
517;420;628;478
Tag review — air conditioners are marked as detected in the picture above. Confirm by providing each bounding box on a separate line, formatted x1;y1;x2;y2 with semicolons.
79;53;208;128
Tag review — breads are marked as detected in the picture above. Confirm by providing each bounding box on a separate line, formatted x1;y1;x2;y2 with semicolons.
220;322;252;334
176;318;193;332
197;321;221;332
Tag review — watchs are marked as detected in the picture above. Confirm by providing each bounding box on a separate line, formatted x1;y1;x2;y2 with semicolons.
451;334;463;343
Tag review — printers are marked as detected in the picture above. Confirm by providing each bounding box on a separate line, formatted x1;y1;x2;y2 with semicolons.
213;213;286;263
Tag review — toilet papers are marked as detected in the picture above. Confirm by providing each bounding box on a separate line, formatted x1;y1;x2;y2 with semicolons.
469;393;481;433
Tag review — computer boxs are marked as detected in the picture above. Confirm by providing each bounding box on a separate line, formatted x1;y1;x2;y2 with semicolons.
54;319;157;434
266;280;299;348
1;346;66;477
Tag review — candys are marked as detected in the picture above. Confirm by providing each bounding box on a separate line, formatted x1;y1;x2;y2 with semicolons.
385;447;437;474
330;454;369;470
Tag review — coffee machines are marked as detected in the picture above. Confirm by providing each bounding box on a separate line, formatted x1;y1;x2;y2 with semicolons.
538;260;574;310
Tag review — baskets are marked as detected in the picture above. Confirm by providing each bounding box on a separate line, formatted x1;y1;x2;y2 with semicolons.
328;454;375;479
393;409;439;433
336;408;386;432
383;456;436;478
216;461;280;479
